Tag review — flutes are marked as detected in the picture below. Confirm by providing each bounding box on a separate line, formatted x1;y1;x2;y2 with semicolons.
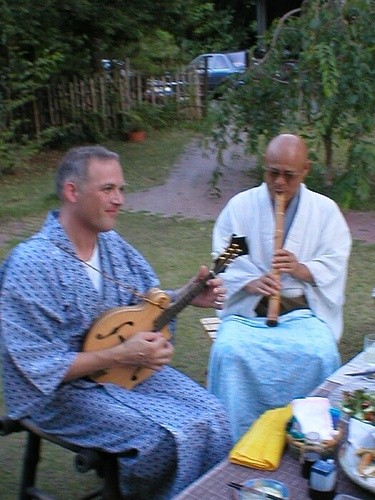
266;193;285;326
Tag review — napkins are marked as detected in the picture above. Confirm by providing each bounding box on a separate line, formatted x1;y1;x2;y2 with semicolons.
229;404;294;471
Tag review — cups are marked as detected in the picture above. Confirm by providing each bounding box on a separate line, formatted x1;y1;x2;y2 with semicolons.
304;431;321;462
363;334;375;365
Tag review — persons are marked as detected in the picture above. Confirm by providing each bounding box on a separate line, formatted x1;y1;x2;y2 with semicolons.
0;145;233;500
207;133;351;445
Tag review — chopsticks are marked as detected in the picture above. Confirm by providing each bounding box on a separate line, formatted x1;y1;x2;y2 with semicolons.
227;482;285;500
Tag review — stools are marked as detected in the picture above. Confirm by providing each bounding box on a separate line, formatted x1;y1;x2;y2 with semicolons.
0;412;137;500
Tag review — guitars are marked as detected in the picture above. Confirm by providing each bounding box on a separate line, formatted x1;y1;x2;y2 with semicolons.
83;234;250;387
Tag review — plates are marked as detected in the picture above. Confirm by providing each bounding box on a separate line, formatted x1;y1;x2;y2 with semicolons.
233;477;289;500
337;439;375;493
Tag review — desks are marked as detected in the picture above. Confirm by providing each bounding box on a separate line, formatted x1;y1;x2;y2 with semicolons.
176;343;375;500
